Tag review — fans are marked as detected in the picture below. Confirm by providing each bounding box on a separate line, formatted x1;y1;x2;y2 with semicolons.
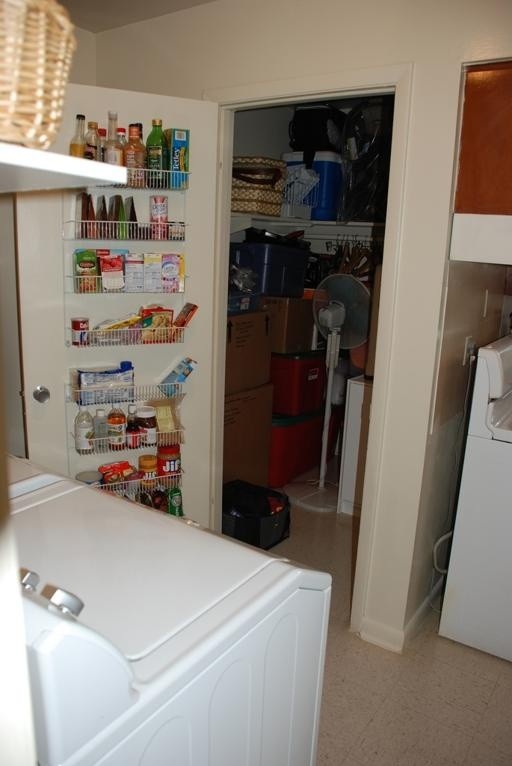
282;274;371;512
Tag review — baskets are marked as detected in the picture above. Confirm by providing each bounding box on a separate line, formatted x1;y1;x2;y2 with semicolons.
231;156;288;216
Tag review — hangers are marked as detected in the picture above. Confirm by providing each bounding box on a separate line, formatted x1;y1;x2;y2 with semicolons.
335;234;369;247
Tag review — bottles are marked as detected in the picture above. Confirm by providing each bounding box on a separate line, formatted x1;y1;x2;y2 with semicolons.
76;471;102;490
159;445;182;487
75;406;158;454
149;195;170;241
70;318;90;346
70;109;169;188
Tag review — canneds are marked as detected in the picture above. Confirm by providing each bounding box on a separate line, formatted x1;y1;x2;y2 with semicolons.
168;222;185;240
72;317;89;346
150;195;168;239
138;444;182;517
137;405;158;448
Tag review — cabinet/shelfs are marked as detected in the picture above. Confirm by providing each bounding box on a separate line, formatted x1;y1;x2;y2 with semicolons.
66;170;191;515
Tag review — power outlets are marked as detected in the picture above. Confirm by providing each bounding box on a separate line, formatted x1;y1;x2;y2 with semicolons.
463;335;475;367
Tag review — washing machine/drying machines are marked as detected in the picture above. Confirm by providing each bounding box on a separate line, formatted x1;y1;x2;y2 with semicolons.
7;450;65;499
10;479;334;766
437;334;512;666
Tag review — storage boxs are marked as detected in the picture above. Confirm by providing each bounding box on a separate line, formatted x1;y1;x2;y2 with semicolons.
271;352;324;416
222;480;290;550
225;311;271;396
269;413;334;488
229;242;241;266
309;254;335;285
281;151;341;221
238;243;310;297
257;297;313;354
222;384;275;489
